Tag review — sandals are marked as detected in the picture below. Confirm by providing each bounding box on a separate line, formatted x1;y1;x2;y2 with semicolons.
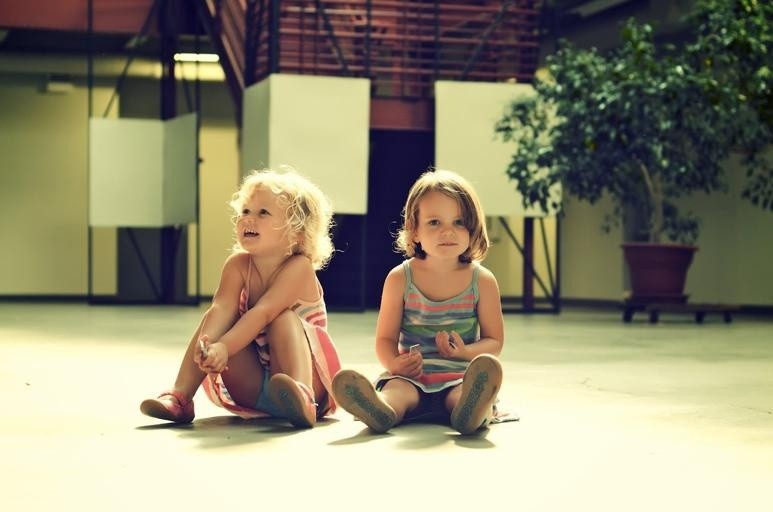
139;390;196;424
328;369;398;433
448;352;503;436
267;373;326;429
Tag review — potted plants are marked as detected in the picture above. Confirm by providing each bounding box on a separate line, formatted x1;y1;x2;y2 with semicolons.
489;0;773;305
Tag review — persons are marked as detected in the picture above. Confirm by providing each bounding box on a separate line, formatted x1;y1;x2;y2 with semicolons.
332;170;518;435
139;164;342;428
